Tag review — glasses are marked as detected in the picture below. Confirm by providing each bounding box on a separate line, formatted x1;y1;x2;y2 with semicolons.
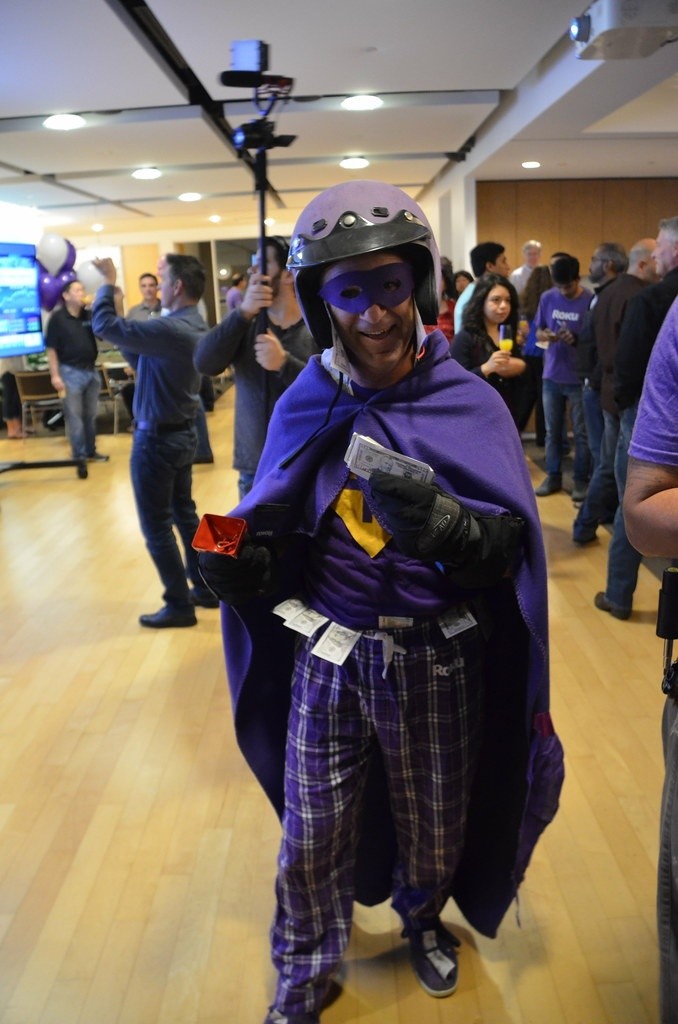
591;257;608;263
252;255;276;266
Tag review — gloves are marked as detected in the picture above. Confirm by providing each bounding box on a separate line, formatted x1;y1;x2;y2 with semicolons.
356;468;467;568
196;529;271;608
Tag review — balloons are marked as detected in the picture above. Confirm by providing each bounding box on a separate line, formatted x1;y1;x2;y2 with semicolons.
36;231;105;313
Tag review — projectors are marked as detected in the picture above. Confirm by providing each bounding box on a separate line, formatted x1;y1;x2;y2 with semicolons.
570;0;678;61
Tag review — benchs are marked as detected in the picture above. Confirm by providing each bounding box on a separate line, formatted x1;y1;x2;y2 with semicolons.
14;364;125;439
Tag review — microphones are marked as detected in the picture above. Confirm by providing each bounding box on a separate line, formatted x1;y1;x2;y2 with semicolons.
220;70;294;88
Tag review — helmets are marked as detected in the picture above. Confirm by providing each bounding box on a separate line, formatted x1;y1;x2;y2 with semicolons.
286;181;440;350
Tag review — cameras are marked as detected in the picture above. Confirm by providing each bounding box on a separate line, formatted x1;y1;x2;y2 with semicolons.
233;122;296;150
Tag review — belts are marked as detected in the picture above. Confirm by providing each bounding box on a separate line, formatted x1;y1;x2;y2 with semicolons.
136;419;195;432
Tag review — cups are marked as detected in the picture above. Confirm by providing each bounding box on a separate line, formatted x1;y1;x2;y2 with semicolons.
499;324;514;352
81;293;97;305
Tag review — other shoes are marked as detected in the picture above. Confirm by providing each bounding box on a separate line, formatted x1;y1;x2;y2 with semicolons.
595;592;631;619
87;451;108;462
76;461;87;479
572;491;582;500
536;472;562;495
575;536;599;547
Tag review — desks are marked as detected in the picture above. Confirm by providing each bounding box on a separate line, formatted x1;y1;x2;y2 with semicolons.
27;360;132;384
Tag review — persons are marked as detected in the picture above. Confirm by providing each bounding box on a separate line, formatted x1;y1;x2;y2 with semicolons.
192;179;566;1024
42;280;110;480
453;270;474;300
423;255;456;345
192;235;319;504
226;273;248;316
623;298;678;1024
454;241;511;338
448;273;536;437
509;218;678;619
91;253;221;629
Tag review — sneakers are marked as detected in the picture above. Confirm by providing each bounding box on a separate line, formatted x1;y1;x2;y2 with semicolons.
401;919;461;997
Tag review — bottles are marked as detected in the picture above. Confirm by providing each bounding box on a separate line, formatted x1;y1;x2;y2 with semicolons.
515;314;530;347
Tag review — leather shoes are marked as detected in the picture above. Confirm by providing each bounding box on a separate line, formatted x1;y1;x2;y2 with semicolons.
194;596;218;609
140;601;197;628
